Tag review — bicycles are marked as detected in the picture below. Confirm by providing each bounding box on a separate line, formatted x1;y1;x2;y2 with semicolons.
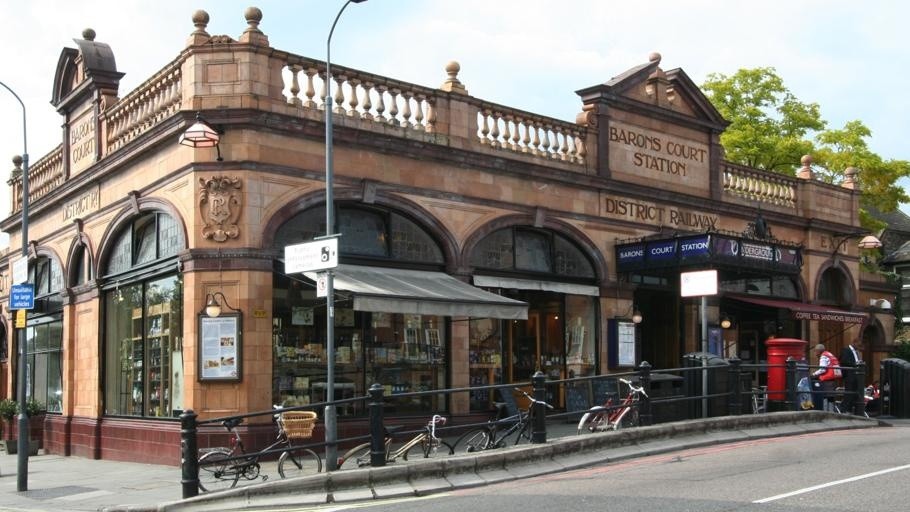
578;378;648;435
197;400;323;493
335;414;455;470
447;388;554;455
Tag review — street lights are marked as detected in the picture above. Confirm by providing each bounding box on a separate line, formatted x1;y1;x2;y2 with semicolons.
325;0;368;472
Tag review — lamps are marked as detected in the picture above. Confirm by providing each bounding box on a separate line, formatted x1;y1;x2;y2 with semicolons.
177;111;225;161
832;231;882;257
196;291;243;319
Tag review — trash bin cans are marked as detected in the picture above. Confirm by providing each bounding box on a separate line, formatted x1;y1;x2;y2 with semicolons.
631;352;730;426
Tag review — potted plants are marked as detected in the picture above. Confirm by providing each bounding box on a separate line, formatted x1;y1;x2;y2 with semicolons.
1;397;43;457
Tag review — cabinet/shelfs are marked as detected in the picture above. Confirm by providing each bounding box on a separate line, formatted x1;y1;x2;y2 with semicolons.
119;298;181;417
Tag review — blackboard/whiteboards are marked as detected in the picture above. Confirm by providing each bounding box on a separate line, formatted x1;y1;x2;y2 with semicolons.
496;388;518;416
564;378;619;422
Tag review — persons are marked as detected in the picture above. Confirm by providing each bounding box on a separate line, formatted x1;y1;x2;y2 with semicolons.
835;338;865;416
812;344;842;410
